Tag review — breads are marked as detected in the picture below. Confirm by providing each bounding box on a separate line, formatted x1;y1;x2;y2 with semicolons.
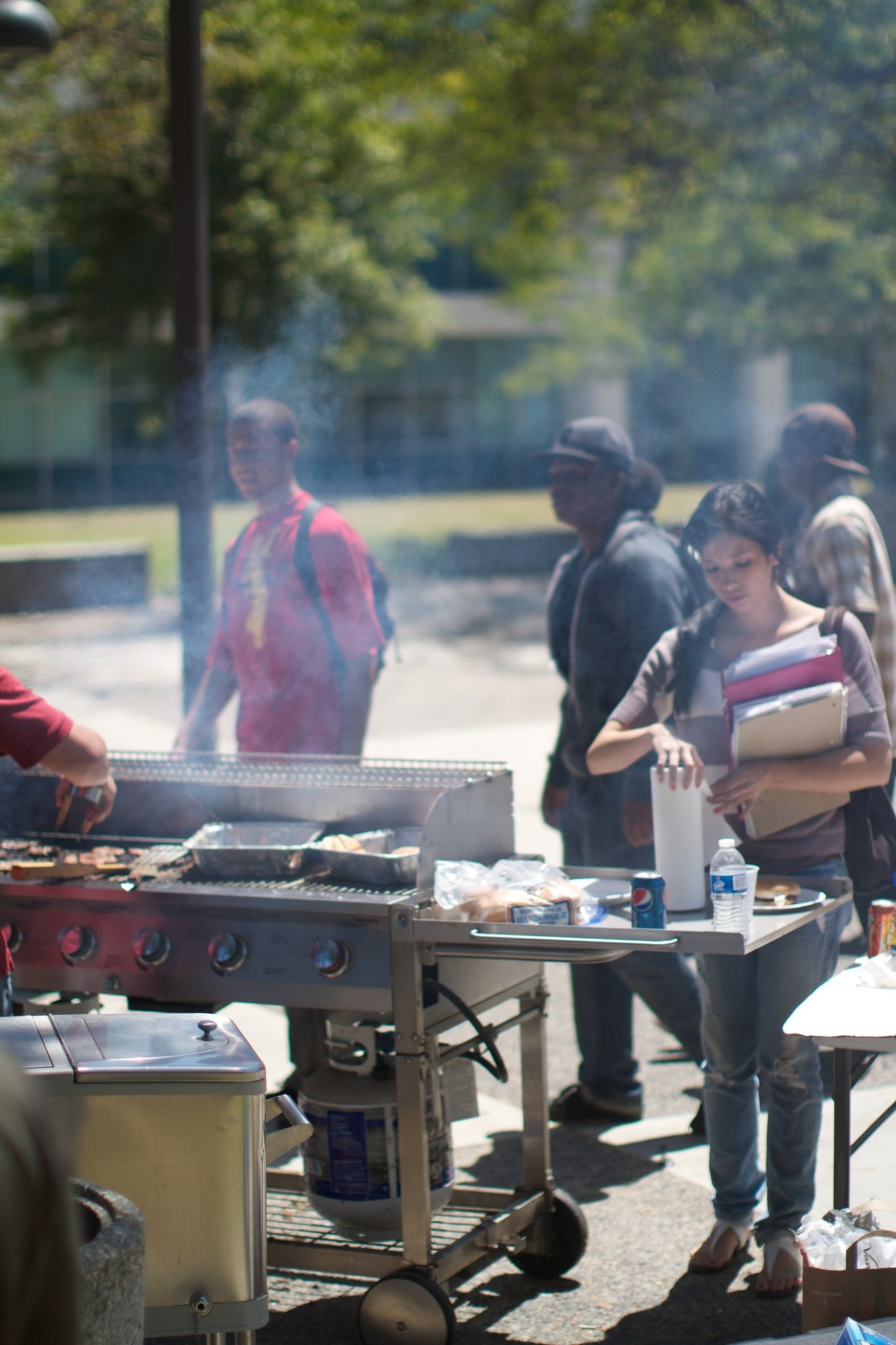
456;880;586;925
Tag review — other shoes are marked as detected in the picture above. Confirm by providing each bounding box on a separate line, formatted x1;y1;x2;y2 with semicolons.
547;1085;634;1121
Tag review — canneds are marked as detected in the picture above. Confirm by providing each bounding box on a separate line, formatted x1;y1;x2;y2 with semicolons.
868;898;896;957
630;870;666;930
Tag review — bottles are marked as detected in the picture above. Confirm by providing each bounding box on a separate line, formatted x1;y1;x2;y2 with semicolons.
710;839;750;943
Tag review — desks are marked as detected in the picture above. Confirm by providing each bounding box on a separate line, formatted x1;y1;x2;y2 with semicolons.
812;950;896;1212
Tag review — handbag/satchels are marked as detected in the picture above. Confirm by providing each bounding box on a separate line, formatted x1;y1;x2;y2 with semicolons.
800;1201;896;1332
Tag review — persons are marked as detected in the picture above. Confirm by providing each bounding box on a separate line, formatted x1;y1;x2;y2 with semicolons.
544;415;710;1133
170;400;394;771
778;402;895;955
0;665;117;1345
587;482;893;1299
764;454;800;584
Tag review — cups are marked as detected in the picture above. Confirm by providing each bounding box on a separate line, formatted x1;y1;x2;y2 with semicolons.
719;865;760;921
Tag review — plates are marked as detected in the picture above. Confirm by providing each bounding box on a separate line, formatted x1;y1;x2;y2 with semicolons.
568;878;631;906
752;886;826;913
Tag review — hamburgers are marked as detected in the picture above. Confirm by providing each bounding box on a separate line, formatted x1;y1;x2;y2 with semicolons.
754;879;801;905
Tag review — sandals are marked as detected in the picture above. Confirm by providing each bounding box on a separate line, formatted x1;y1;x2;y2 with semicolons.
690;1221;753;1272
761;1234;804;1294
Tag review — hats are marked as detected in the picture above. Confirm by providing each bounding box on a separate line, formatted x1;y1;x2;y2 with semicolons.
536;416;633;476
782;400;868;474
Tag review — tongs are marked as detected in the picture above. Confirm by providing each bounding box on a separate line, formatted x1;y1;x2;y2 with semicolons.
53;783;103;845
